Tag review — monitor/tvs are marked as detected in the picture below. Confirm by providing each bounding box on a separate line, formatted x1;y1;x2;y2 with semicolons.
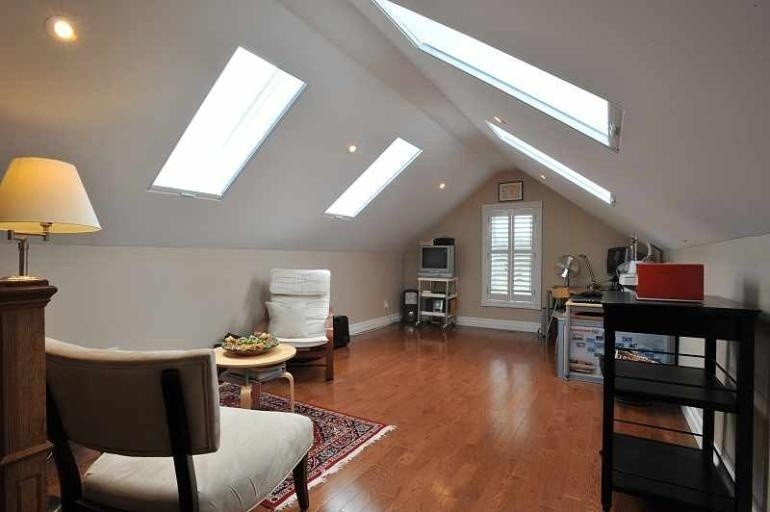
607;246;644;276
418;245;454;278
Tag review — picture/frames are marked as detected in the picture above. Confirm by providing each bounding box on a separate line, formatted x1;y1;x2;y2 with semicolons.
497;180;524;202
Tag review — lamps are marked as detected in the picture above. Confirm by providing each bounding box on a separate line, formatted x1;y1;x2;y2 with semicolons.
1;156;102;281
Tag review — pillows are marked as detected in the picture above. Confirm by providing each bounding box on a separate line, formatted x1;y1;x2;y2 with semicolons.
264;299;308;339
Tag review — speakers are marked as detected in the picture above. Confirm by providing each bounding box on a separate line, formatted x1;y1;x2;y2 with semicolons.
431;291;450;325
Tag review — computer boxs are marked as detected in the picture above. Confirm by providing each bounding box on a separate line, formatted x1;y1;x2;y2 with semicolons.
403;289;418;323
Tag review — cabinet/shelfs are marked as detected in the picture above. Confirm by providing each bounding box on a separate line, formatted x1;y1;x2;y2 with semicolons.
595;290;762;512
415;276;460;330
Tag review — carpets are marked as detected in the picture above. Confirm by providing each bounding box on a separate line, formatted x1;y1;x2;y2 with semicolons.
217;381;398;512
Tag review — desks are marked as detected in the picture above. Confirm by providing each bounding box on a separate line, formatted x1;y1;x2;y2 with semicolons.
544;284;588;344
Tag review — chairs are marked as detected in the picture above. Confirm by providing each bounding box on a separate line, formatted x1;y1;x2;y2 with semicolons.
45;338;316;511
249;269;336;384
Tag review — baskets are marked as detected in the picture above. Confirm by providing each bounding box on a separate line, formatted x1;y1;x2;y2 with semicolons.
222;336;278;356
